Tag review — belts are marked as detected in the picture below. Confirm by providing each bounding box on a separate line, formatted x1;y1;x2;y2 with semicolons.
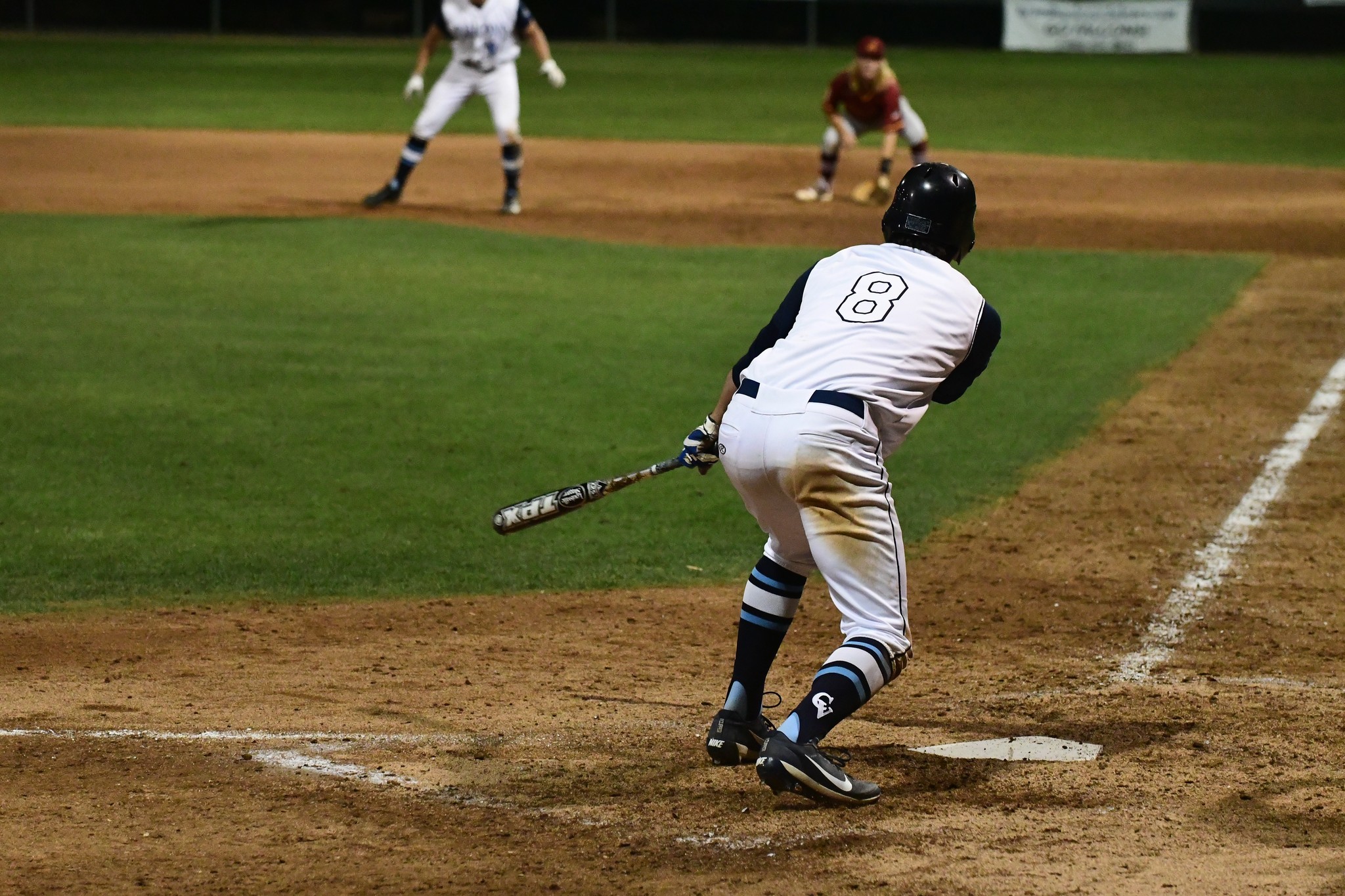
735;377;864;421
464;60;495;73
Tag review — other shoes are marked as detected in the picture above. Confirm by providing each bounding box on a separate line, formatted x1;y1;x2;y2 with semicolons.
795;186;833;202
503;194;522;214
363;186;398;209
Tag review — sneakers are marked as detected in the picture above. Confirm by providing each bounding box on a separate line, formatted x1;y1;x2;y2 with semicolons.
756;731;882;807
706;691;783;767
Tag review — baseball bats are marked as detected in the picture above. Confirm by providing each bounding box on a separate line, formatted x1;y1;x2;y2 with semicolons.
492;443;717;535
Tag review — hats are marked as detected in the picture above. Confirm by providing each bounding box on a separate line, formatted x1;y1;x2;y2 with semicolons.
856;37;885;60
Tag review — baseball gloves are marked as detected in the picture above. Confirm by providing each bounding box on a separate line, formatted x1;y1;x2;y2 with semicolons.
849;174;892;207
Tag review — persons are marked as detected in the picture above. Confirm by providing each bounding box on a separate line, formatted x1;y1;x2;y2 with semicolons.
794;37;928;203
363;0;567;214
681;162;1002;807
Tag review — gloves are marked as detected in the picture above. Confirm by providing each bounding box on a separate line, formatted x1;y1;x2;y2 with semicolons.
404;75;424;101
540;59;565;90
678;413;721;475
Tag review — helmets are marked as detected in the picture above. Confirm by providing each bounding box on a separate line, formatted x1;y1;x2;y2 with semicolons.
883;161;978;259
